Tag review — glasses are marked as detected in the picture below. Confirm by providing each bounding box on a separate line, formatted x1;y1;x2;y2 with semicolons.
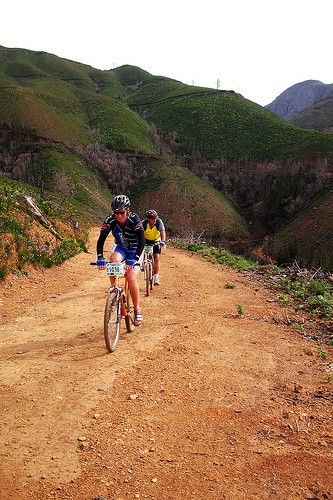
113;211;125;214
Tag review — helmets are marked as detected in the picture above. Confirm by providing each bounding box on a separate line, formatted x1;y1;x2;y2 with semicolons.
110;195;131;211
146;210;158;219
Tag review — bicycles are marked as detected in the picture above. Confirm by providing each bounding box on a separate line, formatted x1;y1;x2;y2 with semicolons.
89;261;142;353
141;241;168;296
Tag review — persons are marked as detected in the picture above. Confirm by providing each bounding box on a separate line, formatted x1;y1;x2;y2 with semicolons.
96;195;145;326
141;210;165;285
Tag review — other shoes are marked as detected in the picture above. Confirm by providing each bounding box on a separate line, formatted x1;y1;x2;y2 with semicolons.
154;273;159;286
133;308;143;326
140;262;145;271
